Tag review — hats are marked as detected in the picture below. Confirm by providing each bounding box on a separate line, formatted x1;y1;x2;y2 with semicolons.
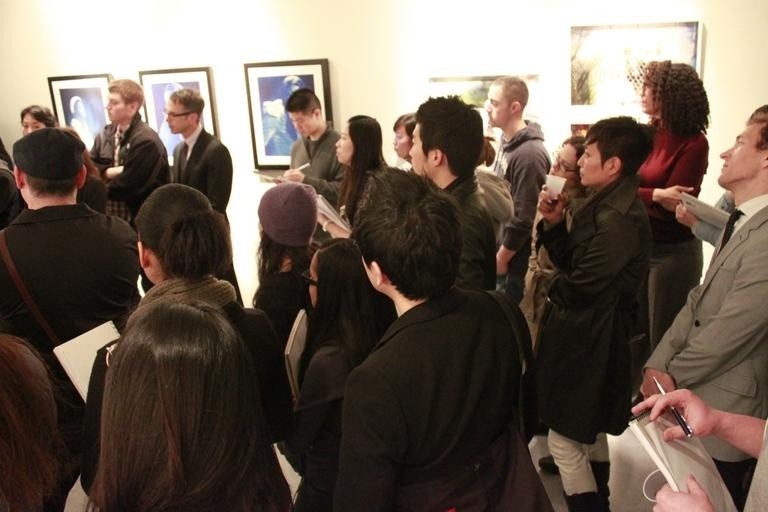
258;182;318;247
12;126;86;180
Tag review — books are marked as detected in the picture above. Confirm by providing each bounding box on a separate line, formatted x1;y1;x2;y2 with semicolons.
54;320;123;405
680;191;730;230
627;404;739;512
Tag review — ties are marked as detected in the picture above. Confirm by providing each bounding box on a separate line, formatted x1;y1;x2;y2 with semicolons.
717;208;744;253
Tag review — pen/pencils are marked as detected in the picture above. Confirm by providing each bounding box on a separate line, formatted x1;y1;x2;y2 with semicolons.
299;162;311;171
653;377;694;438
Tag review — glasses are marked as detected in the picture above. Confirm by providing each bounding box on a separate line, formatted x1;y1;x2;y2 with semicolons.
163;109;191;119
551;150;576;173
300;269;318;289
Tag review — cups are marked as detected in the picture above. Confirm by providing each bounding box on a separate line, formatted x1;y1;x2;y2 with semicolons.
542;172;567;201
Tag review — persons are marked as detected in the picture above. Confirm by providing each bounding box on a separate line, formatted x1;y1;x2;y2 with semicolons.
635;60;707;347
85;185;292;510
671;104;767;248
254;77;655;512
631;387;768;512
0;126;138;510
641;114;768;511
0;333;59;508
21;105;56;134
67;300;288;510
0;133;22;223
59;129;106;212
89;80;167;219
67;94;97;149
169;88;242;310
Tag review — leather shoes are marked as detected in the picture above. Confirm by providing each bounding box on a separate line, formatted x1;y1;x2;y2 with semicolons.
539;455;559;474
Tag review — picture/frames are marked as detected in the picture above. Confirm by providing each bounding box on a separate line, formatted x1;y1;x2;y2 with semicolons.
420;72;542;125
568;19;706;113
240;58;334;171
137;66;219;166
48;72;114;155
